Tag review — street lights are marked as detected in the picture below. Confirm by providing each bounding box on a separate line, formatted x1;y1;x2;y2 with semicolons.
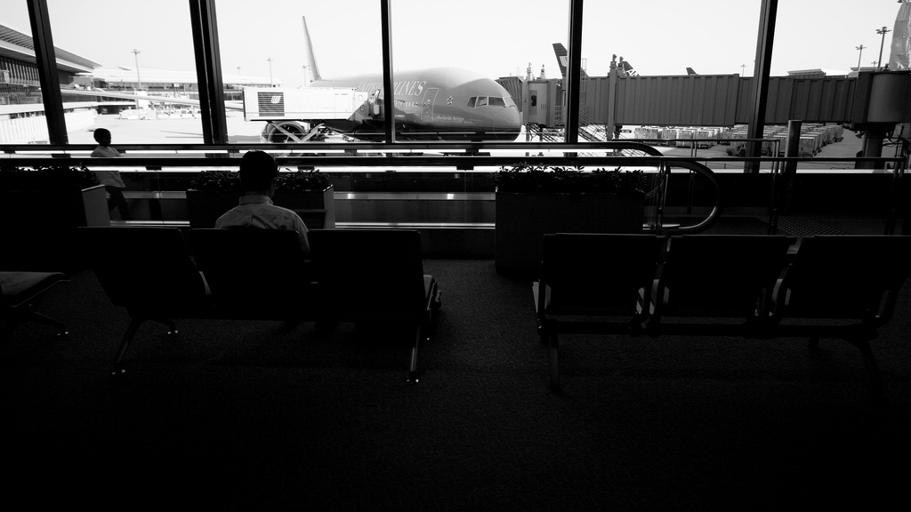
877;25;891;69
131;47;141;87
854;43;866;70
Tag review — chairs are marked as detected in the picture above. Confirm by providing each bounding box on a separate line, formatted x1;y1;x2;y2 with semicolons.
3;222;445;388
492;222;911;387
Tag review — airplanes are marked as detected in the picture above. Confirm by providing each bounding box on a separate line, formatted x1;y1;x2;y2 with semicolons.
60;16;521;141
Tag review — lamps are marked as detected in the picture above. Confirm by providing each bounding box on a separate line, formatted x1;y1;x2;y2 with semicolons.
869;66;911;168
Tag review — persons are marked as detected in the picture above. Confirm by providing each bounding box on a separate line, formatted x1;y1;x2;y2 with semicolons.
90;128;137;222
211;149;311;257
